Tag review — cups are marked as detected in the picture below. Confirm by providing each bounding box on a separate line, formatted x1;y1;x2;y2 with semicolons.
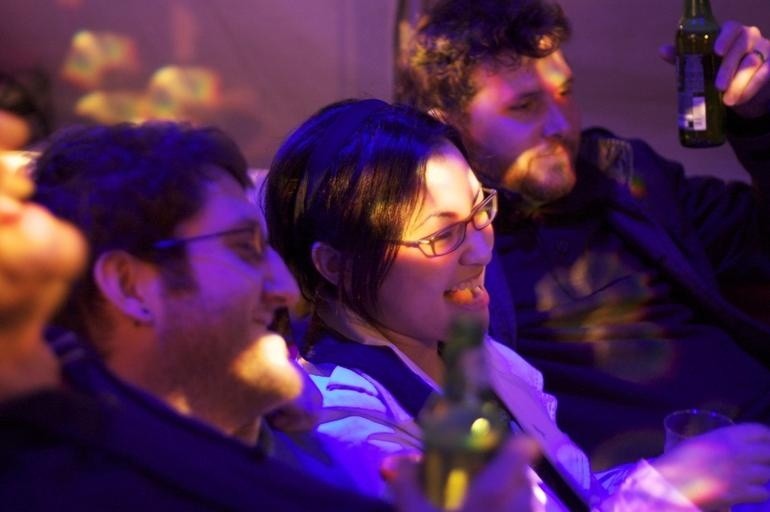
662;409;733;454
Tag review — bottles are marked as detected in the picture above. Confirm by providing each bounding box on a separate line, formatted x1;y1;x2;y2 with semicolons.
421;314;511;509
678;1;725;148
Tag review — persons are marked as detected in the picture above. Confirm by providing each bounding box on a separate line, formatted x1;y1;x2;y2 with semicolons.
2;0;770;512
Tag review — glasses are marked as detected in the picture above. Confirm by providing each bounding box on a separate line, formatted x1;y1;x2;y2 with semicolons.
365;185;500;257
145;225;277;259
